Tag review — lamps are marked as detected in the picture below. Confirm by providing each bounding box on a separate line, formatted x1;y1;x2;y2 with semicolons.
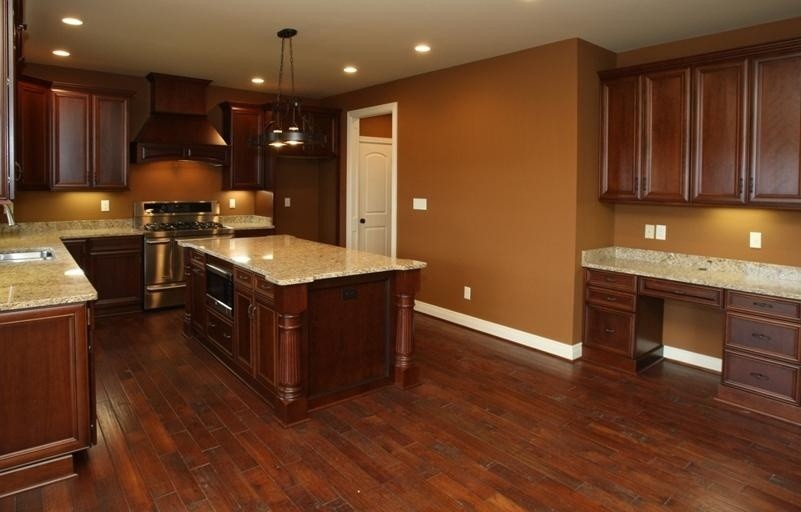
269;29;304;151
750;232;762;247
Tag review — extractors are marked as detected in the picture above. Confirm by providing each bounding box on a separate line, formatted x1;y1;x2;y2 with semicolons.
130;72;232;165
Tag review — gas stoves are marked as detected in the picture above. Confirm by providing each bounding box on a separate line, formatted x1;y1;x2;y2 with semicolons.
141;201;234;237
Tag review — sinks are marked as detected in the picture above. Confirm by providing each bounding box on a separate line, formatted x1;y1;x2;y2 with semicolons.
0;248;56;263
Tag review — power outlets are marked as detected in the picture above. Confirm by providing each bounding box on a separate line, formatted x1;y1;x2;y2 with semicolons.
465;286;472;300
656;225;665;240
645;225;654;239
228;199;235;209
413;198;426;210
102;201;109;210
284;198;290;207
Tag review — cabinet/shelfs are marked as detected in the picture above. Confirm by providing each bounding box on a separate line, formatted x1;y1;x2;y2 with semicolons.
598;55;691;206
583;249;801;429
182;233;428;429
695;39;800;210
221;212;275;234
22;220;144;313
52;86;127;192
270;103;337;160
0;234;92;499
223;99;265;189
14;77;56;196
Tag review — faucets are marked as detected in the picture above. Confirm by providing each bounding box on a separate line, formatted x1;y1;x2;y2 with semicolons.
0;204;18;227
